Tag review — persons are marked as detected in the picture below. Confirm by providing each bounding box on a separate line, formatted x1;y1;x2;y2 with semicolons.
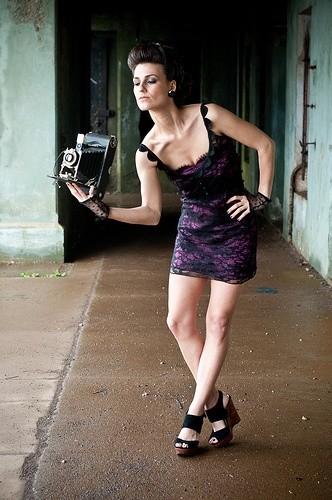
65;43;275;454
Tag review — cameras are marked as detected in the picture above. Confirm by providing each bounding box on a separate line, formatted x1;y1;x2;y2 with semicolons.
48;132;118;199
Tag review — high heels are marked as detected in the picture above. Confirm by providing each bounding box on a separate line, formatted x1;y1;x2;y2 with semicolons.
173;411;207;456
204;390;241;448
